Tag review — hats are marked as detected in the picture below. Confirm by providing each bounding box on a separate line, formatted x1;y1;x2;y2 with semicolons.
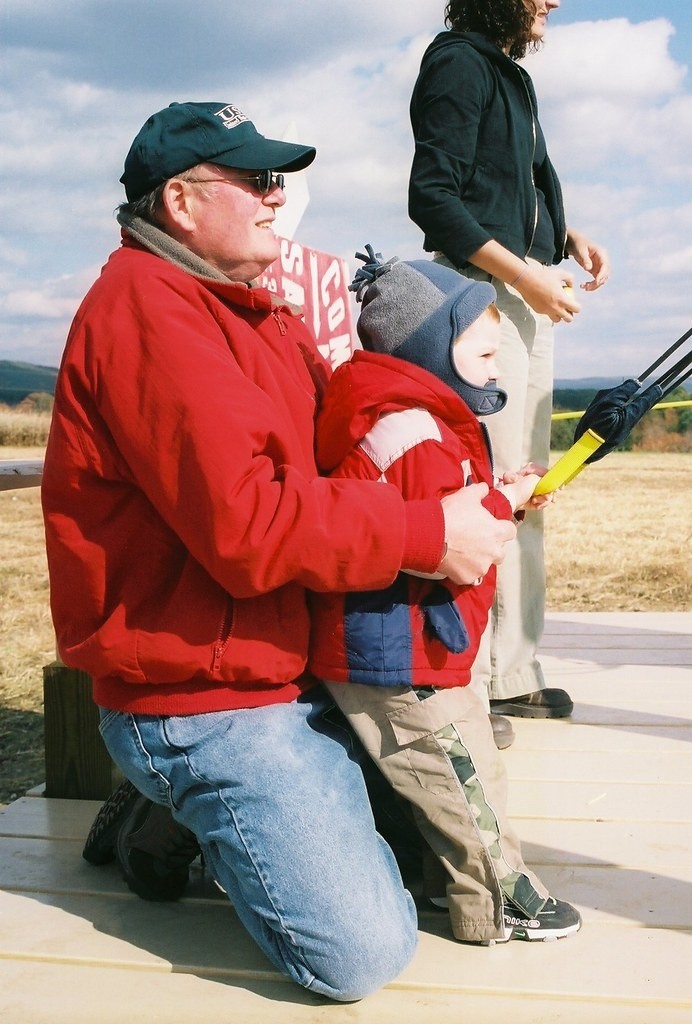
358;256;508;414
121;102;317;203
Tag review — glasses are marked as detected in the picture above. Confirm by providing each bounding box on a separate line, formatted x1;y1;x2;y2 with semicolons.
184;170;288;194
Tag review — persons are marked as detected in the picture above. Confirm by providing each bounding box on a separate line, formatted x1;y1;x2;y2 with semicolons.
307;258;583;943
40;106;513;1006
409;1;609;755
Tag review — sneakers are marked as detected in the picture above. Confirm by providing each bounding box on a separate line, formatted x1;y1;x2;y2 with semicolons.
495;720;512;751
80;781;138;870
478;897;581;947
427;897;447;909
490;688;574;718
120;798;195;904
